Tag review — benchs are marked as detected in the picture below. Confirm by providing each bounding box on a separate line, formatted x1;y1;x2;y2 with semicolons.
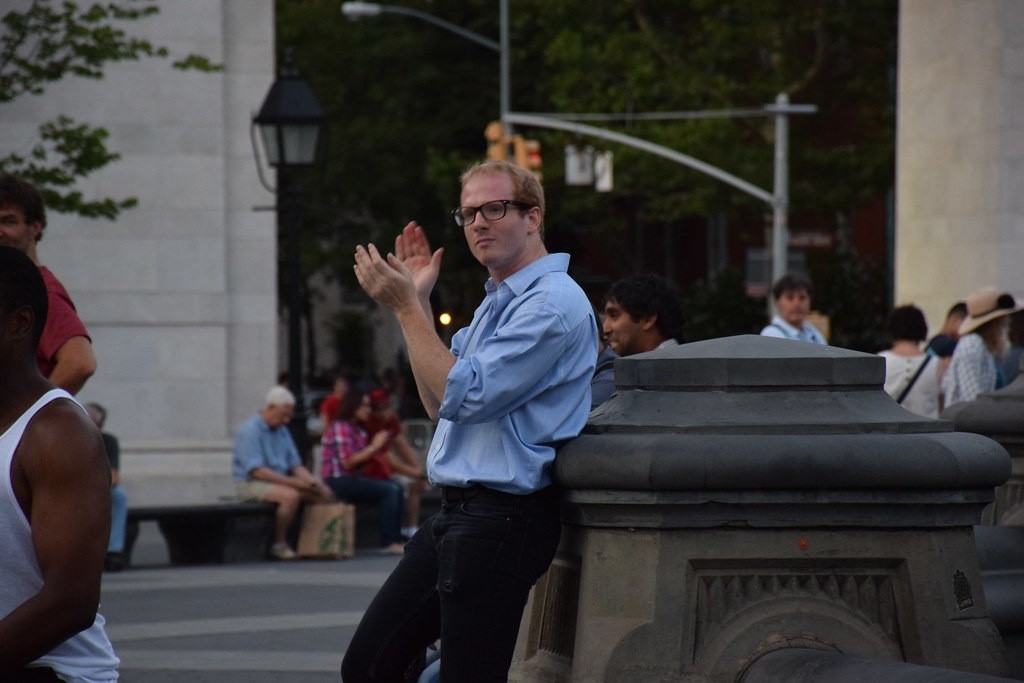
126;500;282;567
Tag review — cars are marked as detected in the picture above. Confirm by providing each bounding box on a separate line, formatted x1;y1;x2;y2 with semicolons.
399;417;439;463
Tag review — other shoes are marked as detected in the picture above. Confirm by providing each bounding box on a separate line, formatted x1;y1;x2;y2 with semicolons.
384;545;404;554
105;560;122;571
271;543;297;559
401;527;418;537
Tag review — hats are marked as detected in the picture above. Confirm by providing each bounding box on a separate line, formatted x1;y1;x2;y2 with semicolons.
370;389;389;403
959;286;1024;333
925;334;957;358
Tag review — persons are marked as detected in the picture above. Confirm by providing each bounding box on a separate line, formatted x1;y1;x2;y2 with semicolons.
229;386;333;560
305;372;426;555
341;161;599;683
601;278;680;360
876;292;1024;420
83;402;127;574
591;311;623;411
0;244;122;683
761;274;828;347
0;176;97;398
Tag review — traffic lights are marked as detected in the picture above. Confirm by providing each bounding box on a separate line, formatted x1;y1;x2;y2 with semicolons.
513;134;544;183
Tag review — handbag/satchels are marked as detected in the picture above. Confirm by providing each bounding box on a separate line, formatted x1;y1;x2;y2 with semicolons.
297;501;354;558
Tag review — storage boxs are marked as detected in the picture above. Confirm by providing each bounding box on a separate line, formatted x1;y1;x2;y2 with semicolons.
297;503;354;558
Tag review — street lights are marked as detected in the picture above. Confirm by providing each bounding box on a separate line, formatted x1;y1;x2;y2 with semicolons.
250;43;339;471
339;1;514;164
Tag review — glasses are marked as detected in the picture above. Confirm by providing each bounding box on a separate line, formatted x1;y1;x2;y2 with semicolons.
376;406;388;411
450;198;529;227
